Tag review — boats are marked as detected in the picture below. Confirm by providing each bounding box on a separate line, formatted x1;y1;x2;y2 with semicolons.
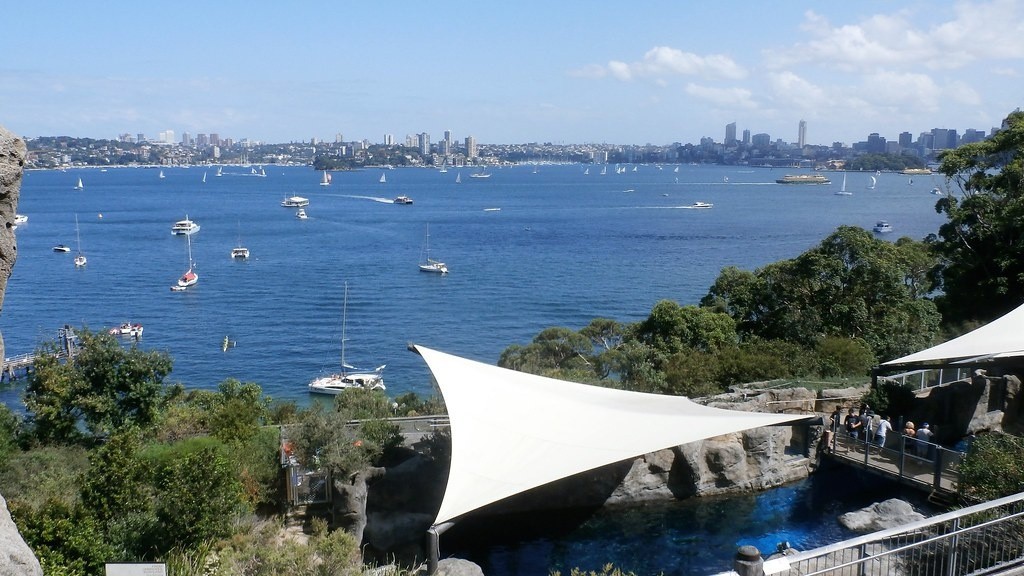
295;208;308;218
171;219;201;236
930;187;941;195
898;167;933;176
170;285;187;291
393;196;414;205
694;201;714;209
52;244;71;252
107;322;143;337
14;214;28;223
872;219;892;233
775;174;832;185
281;192;310;207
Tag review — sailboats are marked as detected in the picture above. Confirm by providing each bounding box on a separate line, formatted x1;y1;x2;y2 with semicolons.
308;282;386;396
866;176;876;190
177;213;199;288
74;213;87;266
73;160;387;190
431;152;701;185
230;219;250;258
833;174;852;196
416;222;450;273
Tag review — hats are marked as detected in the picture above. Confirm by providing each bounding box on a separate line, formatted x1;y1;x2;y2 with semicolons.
923;423;929;425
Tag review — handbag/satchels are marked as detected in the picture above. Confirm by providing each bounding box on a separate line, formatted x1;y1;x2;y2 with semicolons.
873;434;879;442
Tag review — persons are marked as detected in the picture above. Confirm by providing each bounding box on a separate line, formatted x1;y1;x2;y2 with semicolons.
829;405;938;467
